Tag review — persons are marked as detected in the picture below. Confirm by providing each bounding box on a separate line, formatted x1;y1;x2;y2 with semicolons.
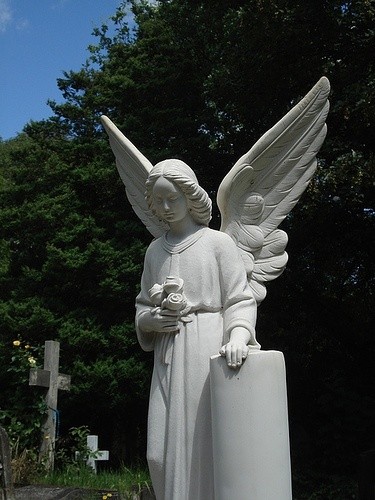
135;157;262;500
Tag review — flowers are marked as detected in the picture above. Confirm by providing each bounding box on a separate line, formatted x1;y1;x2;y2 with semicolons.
147;275;191;367
